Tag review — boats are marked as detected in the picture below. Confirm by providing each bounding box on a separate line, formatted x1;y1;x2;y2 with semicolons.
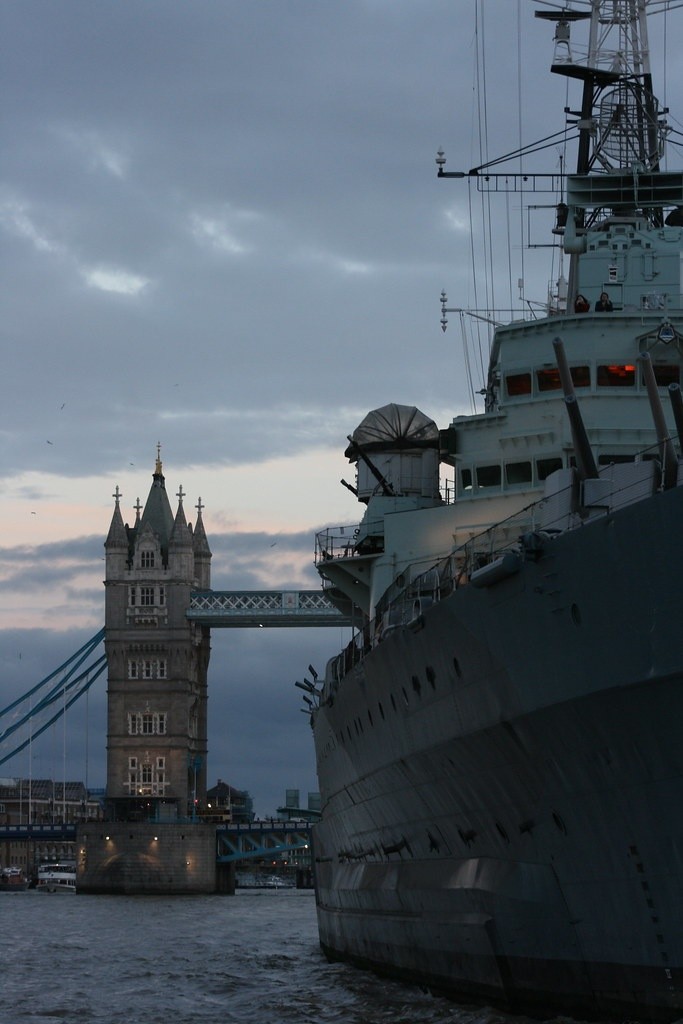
0;867;31;892
38;863;77;894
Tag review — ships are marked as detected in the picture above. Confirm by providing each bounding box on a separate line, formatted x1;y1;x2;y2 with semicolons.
296;2;683;1012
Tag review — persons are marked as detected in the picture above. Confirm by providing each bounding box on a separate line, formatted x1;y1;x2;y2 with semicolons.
594;291;614;313
573;294;591;315
